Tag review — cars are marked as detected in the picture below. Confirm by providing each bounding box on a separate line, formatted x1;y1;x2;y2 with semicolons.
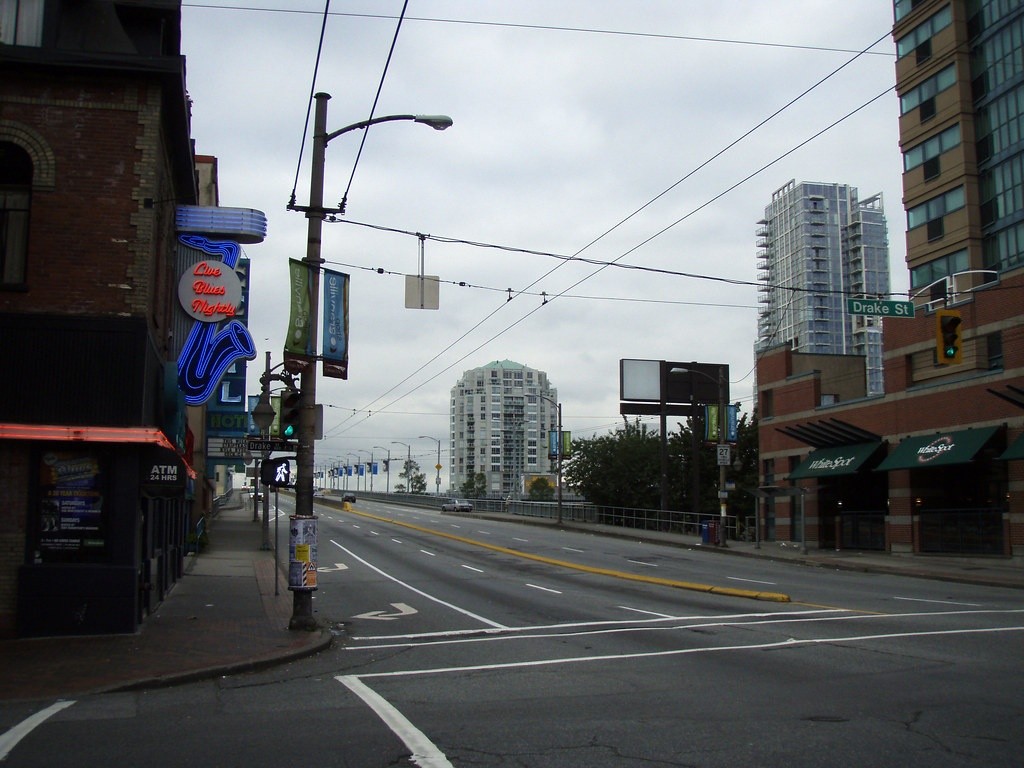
249;488;264;502
442;499;474;513
313;492;324;498
341;493;356;504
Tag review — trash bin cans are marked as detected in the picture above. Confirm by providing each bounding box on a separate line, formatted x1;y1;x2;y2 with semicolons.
702;520;720;544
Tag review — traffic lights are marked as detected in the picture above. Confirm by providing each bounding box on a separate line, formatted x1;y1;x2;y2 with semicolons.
280;391;300;440
937;309;963;365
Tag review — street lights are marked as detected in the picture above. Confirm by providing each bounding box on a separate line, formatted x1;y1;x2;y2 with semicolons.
251;93;453;632
314;449;373;491
418;435;441;497
524;393;561;523
373;446;391;493
390;441;411;494
671;367;727;548
